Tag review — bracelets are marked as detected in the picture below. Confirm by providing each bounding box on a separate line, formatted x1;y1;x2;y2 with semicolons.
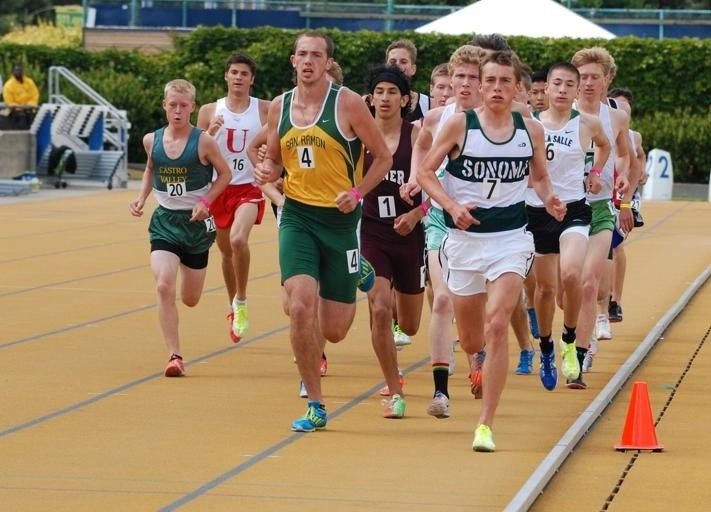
200;198;210;207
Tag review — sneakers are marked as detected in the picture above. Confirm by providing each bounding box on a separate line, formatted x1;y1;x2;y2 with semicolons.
392;320;412;350
232;294;249;338
471;351;485;386
227;311;242;343
516;343;535;375
583;351;594;372
428;389;451;419
560;336;581;380
472;423;496;451
595;313;612;340
165;352;186;377
290;401;327;433
538;337;557;390
567;379;587;388
609;301;622;321
527;307;540;339
383;393;406;418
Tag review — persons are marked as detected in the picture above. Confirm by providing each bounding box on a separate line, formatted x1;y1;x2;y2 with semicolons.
129;80;233;377
2;61;39;116
246;30;648;451
196;53;272;343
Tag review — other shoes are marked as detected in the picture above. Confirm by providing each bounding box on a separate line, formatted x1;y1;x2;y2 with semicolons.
319;353;327;376
300;378;309;397
